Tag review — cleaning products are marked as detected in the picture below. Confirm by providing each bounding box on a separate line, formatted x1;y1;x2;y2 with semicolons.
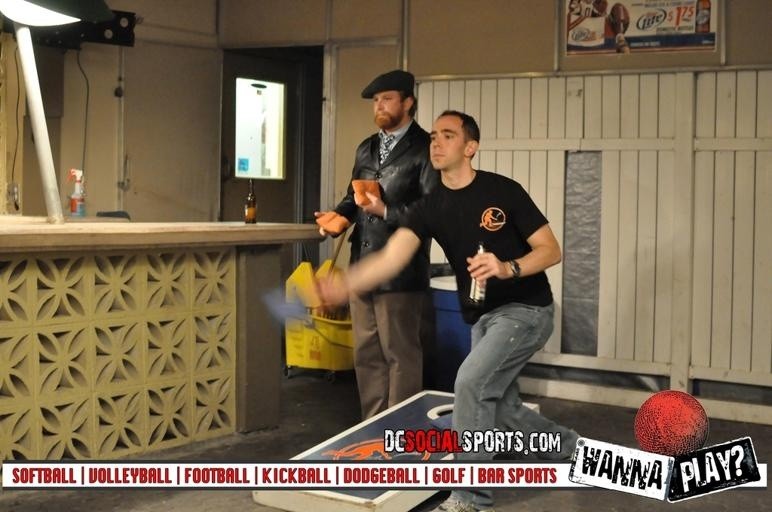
67;167;86;218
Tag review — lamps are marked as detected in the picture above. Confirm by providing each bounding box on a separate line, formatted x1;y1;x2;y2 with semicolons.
0;0;83;28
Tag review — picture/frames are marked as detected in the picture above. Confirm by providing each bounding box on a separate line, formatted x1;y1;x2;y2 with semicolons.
553;0;727;73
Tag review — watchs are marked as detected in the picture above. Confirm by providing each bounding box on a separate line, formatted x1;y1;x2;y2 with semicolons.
509;259;520;278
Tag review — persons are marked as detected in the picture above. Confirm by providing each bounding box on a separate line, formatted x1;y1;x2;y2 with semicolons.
312;108;583;512
312;69;439;422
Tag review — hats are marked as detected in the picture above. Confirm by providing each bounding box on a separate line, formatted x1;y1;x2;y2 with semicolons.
362;71;414;98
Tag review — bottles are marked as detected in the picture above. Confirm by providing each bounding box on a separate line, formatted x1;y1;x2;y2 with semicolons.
468;243;489;312
695;0;712;31
243;178;258;226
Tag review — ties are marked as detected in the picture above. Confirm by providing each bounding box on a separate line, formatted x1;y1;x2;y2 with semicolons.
379;135;394;164
